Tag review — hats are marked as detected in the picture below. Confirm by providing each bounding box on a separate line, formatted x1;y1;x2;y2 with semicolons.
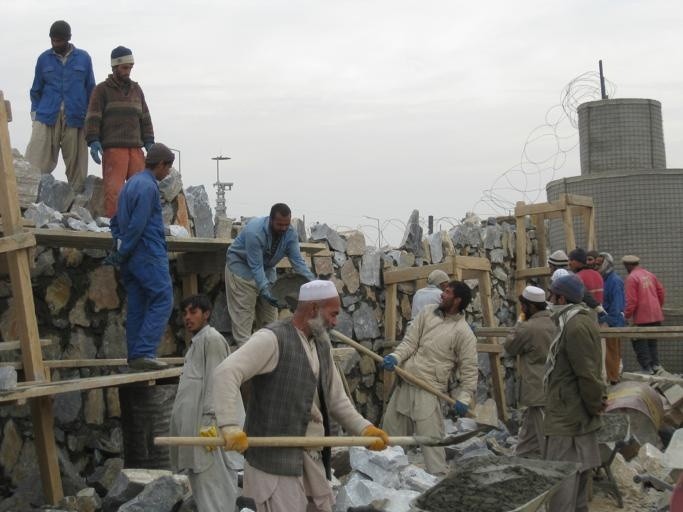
298;280;339;302
427;270;449;286
111;45;135;68
50;21;72;38
145;143;175;164
520;244;640;303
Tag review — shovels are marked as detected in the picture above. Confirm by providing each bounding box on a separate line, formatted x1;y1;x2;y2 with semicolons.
154;429;481;447
268;271;477;417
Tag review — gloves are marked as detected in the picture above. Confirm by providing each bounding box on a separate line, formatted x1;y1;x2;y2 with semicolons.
453;391;473;415
362;425;390;451
380;354;401;371
90;141;104;164
199;425;218;452
222;425;249;454
263;289;280;309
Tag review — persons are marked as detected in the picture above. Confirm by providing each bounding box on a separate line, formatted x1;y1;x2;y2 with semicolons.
502;245;666;512
406;268;451;333
380;280;478;477
166;293;239;512
84;45;155;219
224;202;316;348
29;19;96;194
210;279;391;512
101;142;175;367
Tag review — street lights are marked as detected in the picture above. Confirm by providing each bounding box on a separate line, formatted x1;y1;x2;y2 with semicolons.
209;153;235;218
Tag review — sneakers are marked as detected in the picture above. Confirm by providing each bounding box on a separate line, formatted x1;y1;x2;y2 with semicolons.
129;358;168;370
